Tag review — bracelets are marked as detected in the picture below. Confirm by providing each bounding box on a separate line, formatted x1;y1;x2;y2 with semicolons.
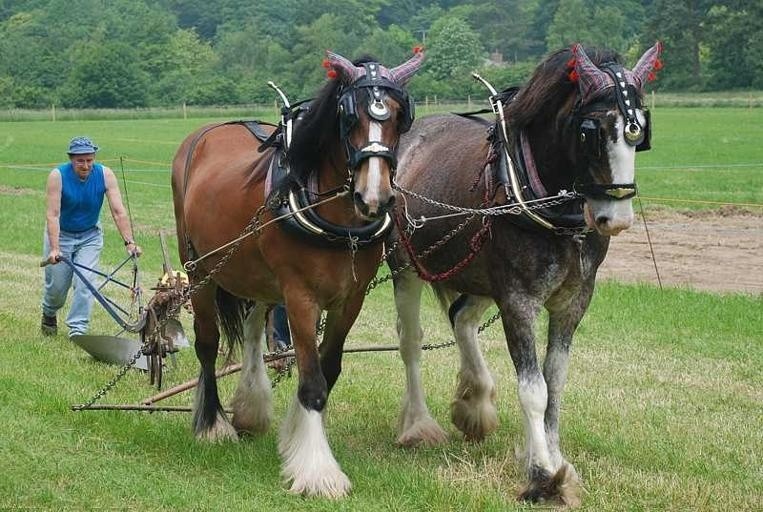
123;240;134;247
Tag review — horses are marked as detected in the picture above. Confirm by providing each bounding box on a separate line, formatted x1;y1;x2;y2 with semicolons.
384;39;662;508
171;49;423;501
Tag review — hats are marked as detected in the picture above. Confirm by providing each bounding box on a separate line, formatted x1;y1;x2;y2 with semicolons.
65;135;99;156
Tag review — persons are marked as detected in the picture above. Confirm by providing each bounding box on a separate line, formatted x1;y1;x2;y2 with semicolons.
37;135;141;340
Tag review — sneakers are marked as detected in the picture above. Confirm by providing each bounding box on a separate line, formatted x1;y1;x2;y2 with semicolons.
40;311;57;337
